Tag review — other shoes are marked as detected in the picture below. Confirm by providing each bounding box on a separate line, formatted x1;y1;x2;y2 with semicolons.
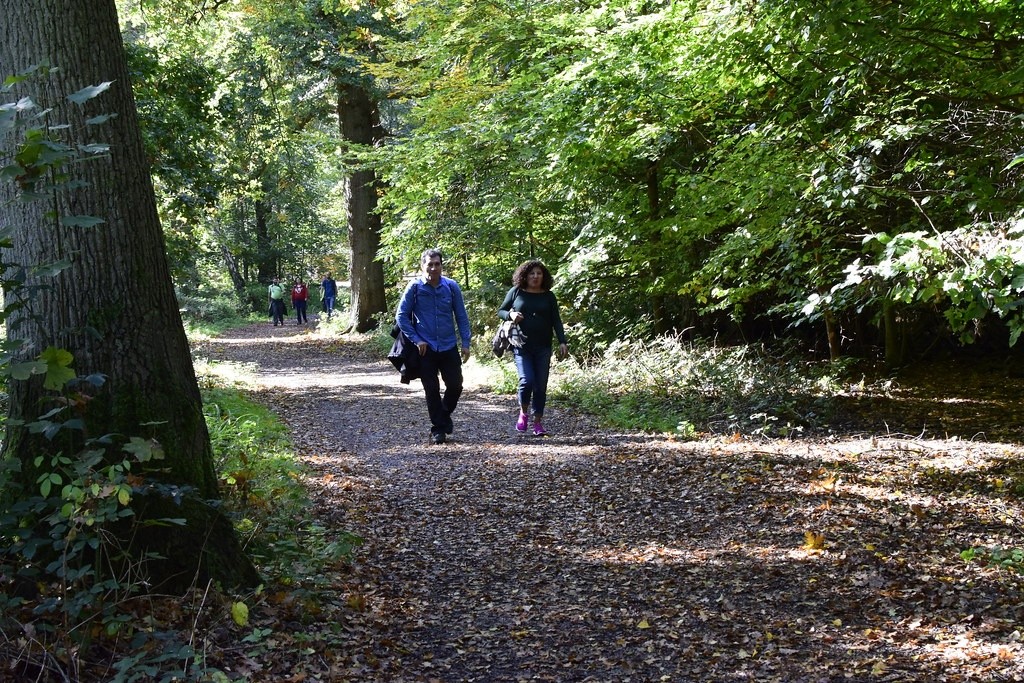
274;323;277;326
442;415;453;434
431;425;445;443
305;321;308;325
297;322;301;325
281;322;283;326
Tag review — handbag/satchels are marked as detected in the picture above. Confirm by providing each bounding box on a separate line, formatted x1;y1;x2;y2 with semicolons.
390;323;400;339
498;337;508;349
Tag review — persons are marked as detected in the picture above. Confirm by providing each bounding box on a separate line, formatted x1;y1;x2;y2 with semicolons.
268;276;286;326
290;277;308;325
498;260;567;435
396;249;471;444
321;271;337;322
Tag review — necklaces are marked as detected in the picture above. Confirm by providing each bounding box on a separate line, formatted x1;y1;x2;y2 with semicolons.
526;288;541;316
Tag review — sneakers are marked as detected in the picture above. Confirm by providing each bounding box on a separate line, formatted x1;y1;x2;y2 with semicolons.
533;421;547;436
516;411;528;433
